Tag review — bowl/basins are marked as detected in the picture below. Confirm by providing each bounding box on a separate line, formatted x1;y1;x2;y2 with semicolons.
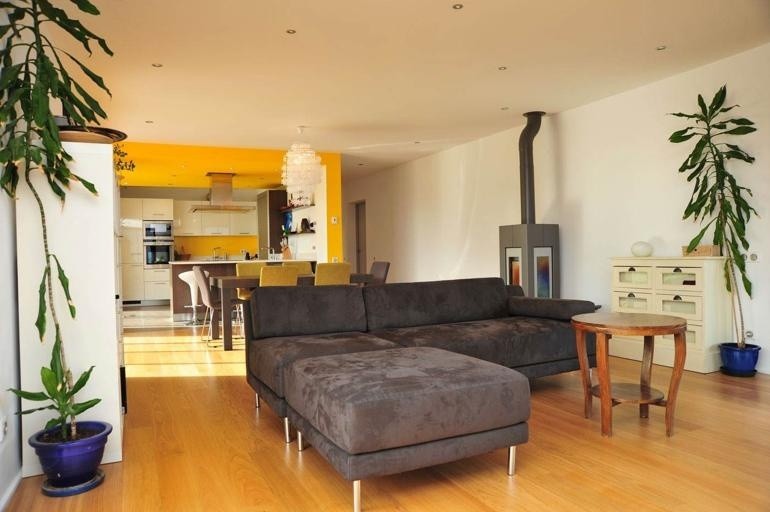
177;253;191;260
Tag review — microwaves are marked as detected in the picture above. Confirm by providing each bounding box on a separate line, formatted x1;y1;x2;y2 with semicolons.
144;220;173;241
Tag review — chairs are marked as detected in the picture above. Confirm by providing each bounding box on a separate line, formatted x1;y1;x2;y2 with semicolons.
236;262;267;324
259;265;297;287
282;262;313;274
364;261;390;285
192;265;247;346
314;263;350;285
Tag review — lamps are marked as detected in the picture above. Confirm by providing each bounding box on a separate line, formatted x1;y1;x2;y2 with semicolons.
281;143;323;206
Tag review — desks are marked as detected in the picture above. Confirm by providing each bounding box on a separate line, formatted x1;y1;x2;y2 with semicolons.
208;272;373;351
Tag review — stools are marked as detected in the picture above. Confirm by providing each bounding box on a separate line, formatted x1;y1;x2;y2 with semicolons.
177;270;210;326
282;347;533;512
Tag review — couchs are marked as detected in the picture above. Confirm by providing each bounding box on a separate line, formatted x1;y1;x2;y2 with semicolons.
243;277;595;442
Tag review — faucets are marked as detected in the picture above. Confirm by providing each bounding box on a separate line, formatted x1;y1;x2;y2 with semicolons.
213;247;221;260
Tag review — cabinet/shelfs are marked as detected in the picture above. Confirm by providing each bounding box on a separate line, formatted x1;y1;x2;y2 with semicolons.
174;200;259;236
608;254;734;374
279;205;315;235
121;198;173;300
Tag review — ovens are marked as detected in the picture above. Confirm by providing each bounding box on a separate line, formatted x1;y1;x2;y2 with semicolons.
144;242;175;269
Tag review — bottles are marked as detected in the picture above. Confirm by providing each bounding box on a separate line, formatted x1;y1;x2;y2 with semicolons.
301;218;309;233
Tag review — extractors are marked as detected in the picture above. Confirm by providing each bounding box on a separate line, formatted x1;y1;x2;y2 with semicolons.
187;183;256;216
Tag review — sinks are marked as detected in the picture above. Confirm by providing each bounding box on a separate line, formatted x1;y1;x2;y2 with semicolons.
200;260;243;261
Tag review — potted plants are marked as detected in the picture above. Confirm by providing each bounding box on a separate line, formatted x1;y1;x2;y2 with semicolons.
666;84;763;377
0;0;113;497
